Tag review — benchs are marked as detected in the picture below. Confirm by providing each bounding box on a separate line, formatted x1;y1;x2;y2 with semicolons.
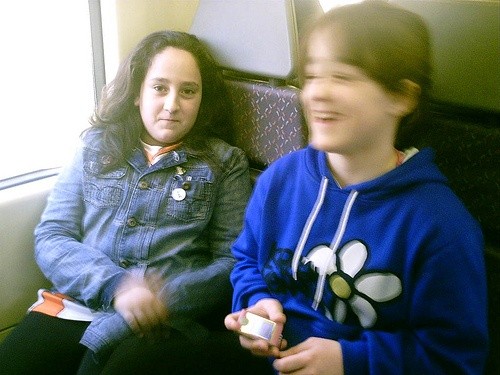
221;78;500;375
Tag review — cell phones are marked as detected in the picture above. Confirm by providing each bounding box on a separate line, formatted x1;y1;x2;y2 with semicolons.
237;309;284;347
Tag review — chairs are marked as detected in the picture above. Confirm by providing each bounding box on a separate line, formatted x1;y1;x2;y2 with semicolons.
190;1;325;84
370;0;500;114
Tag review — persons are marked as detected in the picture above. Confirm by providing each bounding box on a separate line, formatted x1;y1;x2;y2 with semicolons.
0;30;252;375
100;0;489;375
